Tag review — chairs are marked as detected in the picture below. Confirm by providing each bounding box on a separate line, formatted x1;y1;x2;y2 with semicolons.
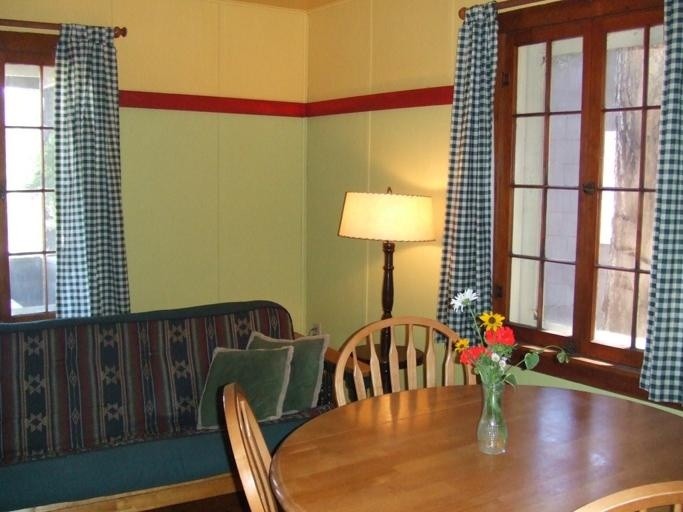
573;480;682;512
332;316;477;408
222;382;282;512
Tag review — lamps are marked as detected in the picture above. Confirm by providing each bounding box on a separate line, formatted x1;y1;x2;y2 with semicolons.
336;186;437;394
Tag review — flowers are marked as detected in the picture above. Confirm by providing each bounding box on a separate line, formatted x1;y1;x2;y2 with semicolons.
449;287;571;444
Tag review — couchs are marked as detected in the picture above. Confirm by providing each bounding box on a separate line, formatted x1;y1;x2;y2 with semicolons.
0;300;371;512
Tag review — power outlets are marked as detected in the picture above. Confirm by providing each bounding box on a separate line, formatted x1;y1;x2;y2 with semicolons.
312;323;321;335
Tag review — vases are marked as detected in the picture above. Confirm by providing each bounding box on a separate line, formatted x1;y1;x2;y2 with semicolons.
476;382;508;456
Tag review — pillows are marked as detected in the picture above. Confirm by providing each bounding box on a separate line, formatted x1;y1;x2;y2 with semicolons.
244;330;330;416
196;345;294;431
